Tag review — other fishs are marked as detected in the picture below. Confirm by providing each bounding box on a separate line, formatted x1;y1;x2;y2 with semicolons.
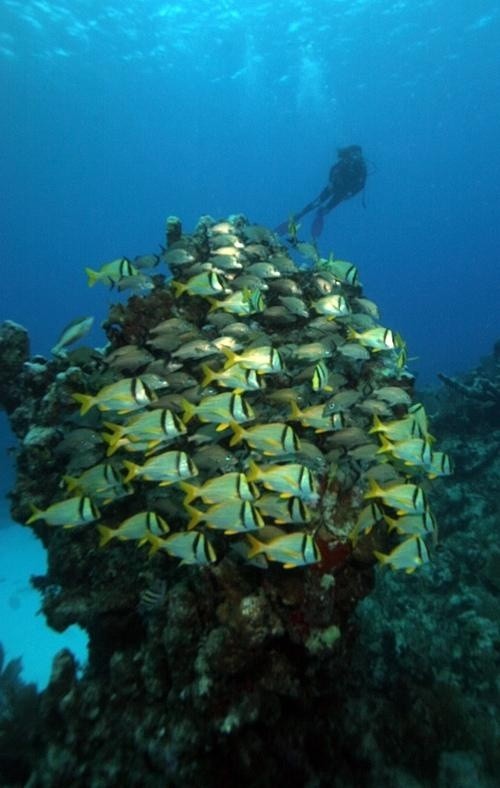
24;221;439;576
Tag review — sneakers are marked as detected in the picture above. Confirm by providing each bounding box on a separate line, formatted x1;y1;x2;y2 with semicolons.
290;215;300;223
316;207;327;216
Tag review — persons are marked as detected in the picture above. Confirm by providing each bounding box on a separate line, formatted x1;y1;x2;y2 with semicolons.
272;146;366;240
